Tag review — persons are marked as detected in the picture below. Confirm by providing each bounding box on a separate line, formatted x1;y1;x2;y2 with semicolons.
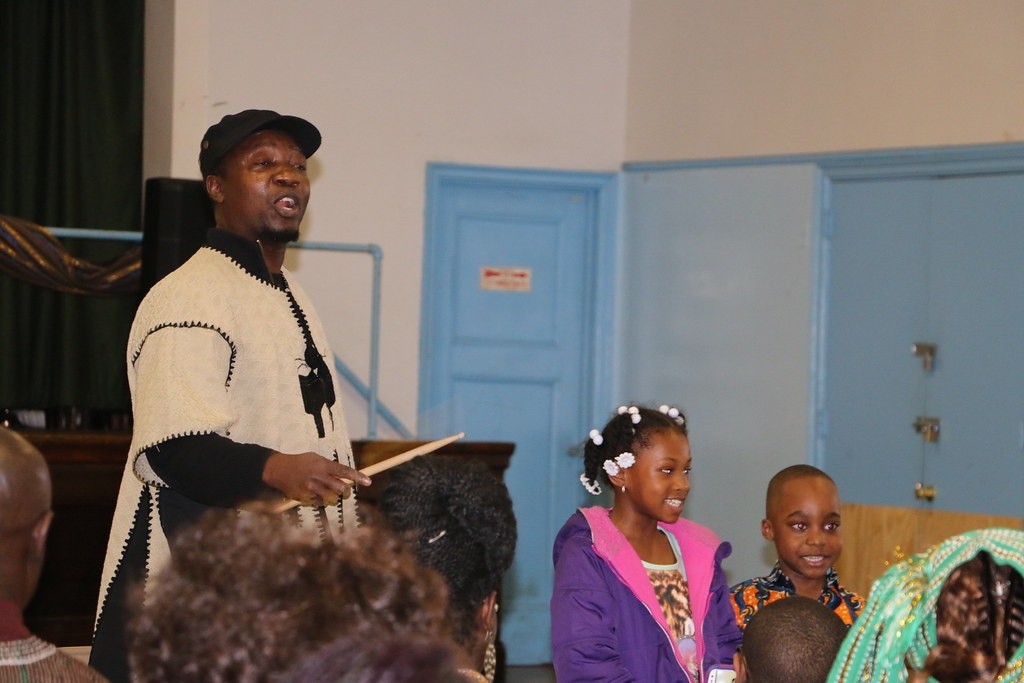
1;426;112;683
138;507;457;681
273;628;492;683
732;595;849;683
550;405;742;683
902;550;1024;683
362;456;518;675
87;108;374;682
728;464;867;646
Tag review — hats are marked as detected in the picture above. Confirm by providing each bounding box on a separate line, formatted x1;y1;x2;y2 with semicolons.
194;102;326;179
